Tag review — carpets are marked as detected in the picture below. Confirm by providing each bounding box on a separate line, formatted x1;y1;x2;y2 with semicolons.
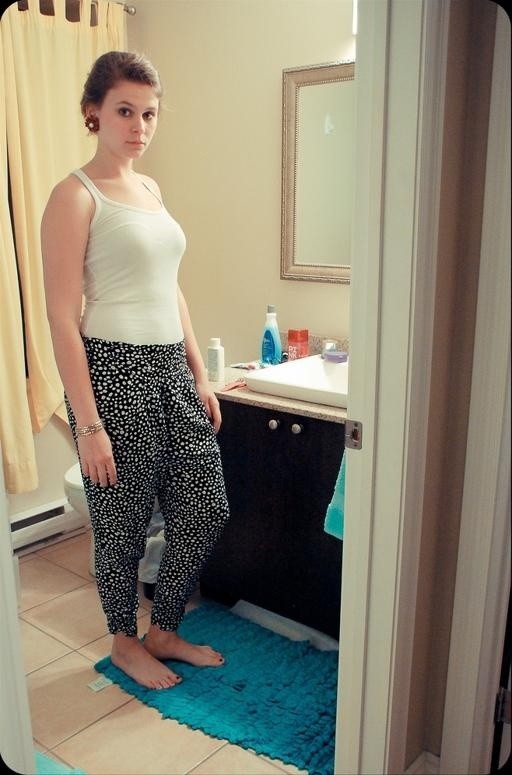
94;605;337;775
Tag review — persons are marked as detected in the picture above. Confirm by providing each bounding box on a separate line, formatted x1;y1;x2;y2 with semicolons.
37;50;233;691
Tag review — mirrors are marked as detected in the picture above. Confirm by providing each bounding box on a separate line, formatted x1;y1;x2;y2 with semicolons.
279;55;356;285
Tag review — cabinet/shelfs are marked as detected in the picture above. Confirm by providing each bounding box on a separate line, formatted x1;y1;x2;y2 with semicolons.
201;398;345;644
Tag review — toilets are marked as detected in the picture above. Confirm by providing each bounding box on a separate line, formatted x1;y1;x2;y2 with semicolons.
63;461;96;577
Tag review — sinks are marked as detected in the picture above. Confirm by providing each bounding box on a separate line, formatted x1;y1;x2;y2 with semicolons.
244;352;347;408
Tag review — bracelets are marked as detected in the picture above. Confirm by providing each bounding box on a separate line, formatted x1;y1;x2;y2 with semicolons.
74;421;104;438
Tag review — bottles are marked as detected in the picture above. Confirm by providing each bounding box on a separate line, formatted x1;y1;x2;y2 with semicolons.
206;337;227;392
261;304;282;366
286;329;309;359
